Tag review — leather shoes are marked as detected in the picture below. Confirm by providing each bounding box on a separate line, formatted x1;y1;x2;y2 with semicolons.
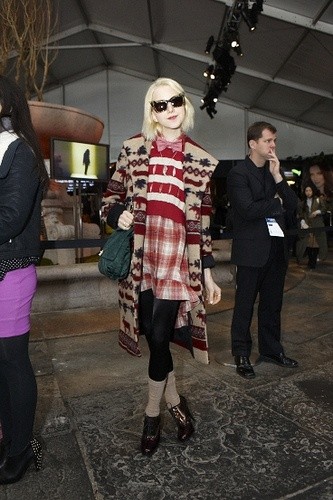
235;355;256;379
261;352;299;367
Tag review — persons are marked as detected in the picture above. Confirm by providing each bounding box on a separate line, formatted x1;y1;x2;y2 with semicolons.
82;149;90;175
99;77;222;457
300;186;327;269
0;74;49;485
227;122;299;379
307;161;333;210
83;195;97;223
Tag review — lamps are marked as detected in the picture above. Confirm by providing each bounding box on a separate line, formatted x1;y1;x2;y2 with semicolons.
198;0;264;120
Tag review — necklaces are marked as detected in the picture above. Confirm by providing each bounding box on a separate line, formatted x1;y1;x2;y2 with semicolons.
159;149;177;175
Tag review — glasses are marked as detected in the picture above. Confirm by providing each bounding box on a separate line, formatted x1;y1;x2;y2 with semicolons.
150;92;185;113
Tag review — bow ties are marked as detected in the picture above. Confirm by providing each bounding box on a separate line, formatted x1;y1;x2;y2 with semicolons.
156;136;182;152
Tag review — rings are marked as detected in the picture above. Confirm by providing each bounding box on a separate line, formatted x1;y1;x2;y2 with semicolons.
123;222;126;225
218;295;221;297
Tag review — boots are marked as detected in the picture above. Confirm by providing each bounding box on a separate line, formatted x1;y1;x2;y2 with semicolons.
306;246;319;269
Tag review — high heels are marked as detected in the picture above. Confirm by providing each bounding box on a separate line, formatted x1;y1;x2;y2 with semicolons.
165;394;196;441
141;408;163;455
0;435;41;485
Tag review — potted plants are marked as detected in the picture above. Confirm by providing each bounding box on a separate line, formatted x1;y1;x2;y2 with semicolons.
0;0;104;158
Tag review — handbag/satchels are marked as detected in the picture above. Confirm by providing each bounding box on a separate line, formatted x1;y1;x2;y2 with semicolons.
98;226;134;280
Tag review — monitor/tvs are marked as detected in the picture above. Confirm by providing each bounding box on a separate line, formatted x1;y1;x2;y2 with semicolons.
50;138;111;183
